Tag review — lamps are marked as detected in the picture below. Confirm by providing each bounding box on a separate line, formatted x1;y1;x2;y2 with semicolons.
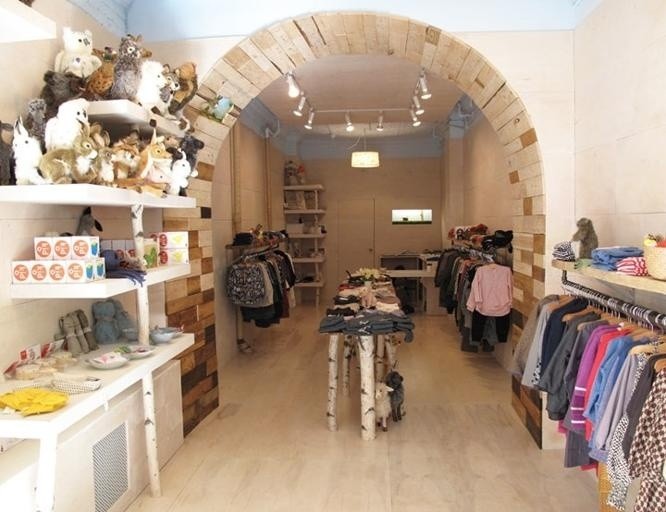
284;69;317;132
342;110;387;133
408;71;433;128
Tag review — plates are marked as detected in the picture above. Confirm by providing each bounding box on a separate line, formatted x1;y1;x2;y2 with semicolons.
84;360;130;368
120;345;157;357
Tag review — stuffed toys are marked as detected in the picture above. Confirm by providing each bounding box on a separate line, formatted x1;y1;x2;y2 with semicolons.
0;23;204;197
93;247;149;288
572;218;599;259
106;299;139;341
57;206;104;239
90;300;116;345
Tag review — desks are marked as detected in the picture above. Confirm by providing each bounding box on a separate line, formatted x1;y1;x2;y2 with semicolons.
378;251;449;316
322;267;410;438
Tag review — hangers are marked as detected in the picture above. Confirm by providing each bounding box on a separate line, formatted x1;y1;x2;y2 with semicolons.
452;244;500;260
559;279;666;338
239;245;281;263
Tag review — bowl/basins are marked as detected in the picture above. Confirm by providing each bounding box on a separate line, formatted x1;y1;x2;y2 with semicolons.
123;326;182;343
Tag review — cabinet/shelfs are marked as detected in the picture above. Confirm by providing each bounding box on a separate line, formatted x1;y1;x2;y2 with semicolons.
282;184;327;309
0;96;197;512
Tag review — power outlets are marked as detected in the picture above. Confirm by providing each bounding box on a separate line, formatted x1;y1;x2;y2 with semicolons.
351;127;381;168
